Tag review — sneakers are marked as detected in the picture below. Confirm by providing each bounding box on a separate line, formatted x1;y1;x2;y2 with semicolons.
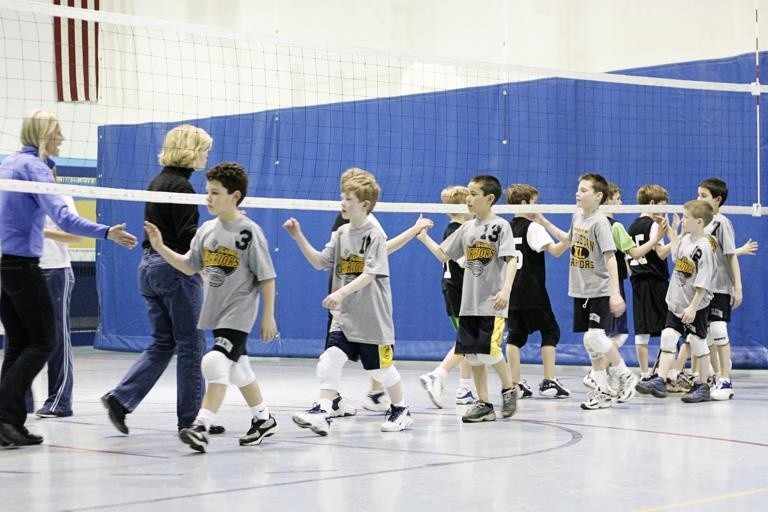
36;408;73;417
177;421;225;451
581;370;734;410
241;415;277;446
295;389;413;435
420;372;572;423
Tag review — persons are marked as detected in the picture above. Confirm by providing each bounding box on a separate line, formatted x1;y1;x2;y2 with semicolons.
527;173;758;410
143;161;281;453
99;120;248;438
25;164;79;422
285;167;434;436
0;108;137;451
415;174;574;422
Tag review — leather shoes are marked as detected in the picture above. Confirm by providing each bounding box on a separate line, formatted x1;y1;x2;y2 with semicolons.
102;392;128;434
0;422;43;445
